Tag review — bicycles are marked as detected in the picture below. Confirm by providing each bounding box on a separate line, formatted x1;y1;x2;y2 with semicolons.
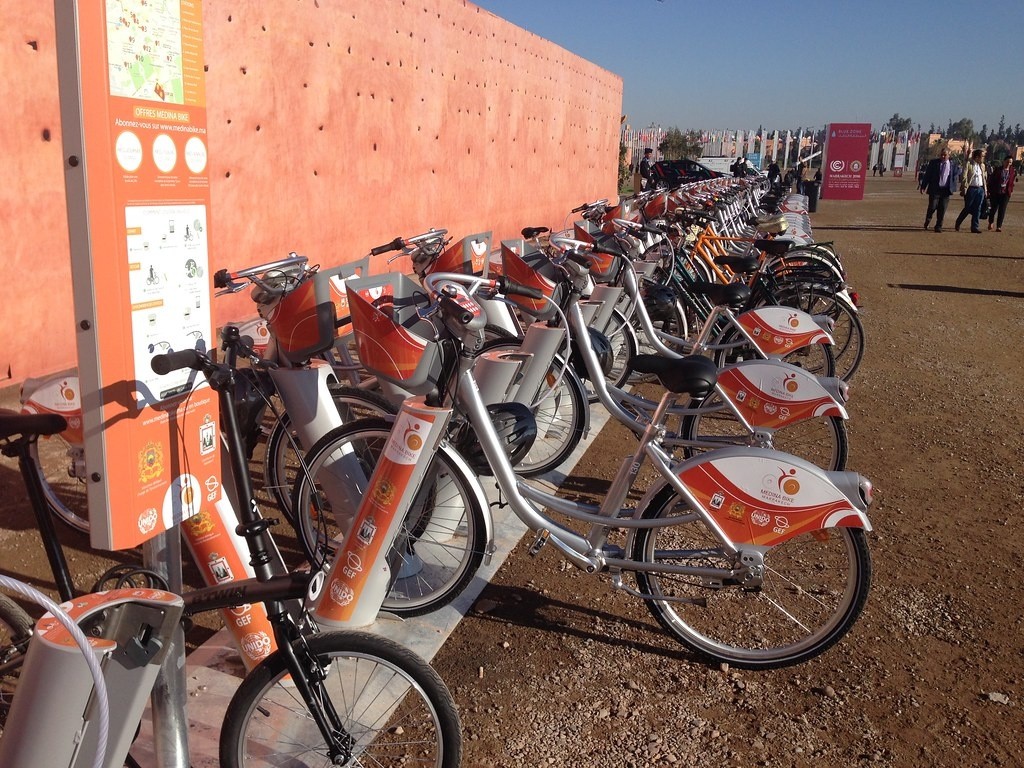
0;173;873;767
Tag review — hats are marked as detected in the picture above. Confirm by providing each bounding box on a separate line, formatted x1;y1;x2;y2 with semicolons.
645;148;653;153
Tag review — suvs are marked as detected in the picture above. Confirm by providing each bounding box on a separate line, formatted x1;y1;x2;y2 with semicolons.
648;160;733;193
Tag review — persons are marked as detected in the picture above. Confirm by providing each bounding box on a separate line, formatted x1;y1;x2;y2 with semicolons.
872;164;878;177
987;155;1018;232
690;164;696;172
919;148;959;233
878;162;884;177
955;149;989;233
732;156;743;178
640;147;655;191
739;157;749;177
915;154;1018;194
764;151;823;214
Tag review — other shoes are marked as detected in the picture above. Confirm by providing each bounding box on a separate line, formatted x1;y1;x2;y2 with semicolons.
971;229;982;233
955;221;960;231
924;222;929;229
996;228;1001;232
988;223;993;230
934;226;942;232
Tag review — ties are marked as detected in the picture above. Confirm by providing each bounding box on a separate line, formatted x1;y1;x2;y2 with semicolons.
940;162;947;179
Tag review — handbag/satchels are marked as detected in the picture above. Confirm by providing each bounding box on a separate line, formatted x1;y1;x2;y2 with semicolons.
979;198;992;220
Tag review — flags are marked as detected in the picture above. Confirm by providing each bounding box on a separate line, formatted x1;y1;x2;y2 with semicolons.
620;121;921;154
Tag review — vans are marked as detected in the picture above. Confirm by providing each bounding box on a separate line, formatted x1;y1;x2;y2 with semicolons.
695;157;758;175
